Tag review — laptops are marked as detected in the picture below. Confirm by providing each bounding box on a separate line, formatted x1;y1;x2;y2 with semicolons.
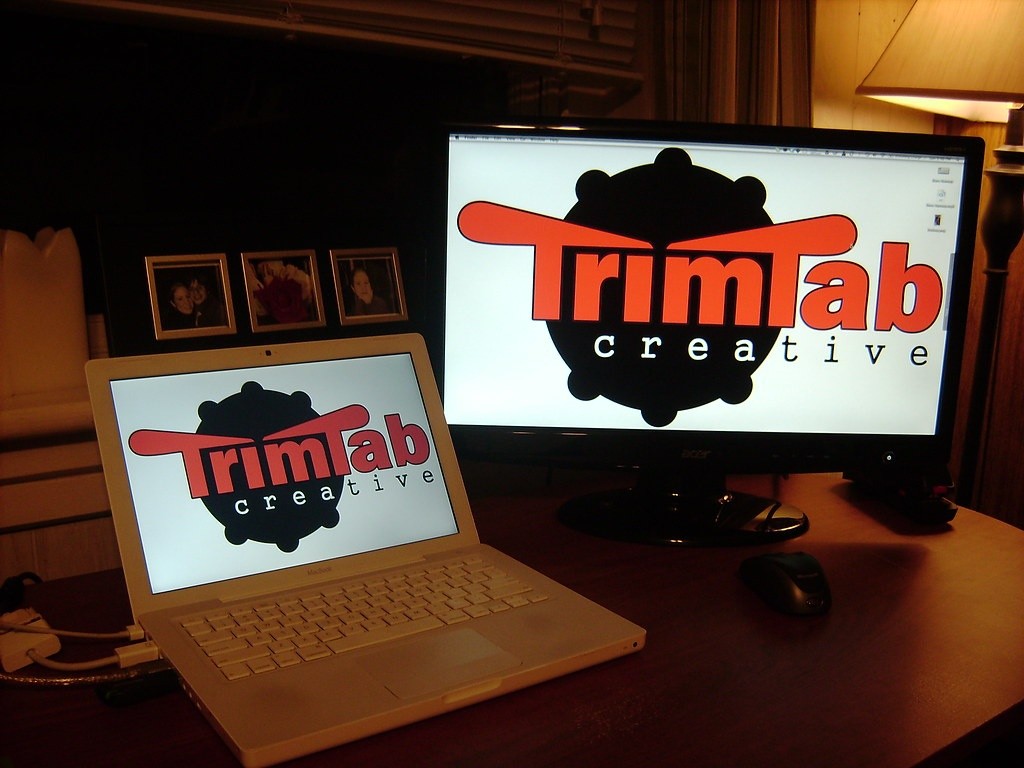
86;331;647;768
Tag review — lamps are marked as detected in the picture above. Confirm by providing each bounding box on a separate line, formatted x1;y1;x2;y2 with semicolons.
856;0;1024;511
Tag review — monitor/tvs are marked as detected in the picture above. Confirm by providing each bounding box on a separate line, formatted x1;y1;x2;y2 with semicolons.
428;119;985;545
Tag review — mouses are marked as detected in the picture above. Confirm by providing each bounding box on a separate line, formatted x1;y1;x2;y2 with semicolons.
738;552;833;619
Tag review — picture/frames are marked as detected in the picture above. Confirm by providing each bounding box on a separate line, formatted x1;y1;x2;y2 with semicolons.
240;249;326;333
329;246;409;327
144;252;238;340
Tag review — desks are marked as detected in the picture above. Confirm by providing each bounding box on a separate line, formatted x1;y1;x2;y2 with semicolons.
0;470;1024;768
